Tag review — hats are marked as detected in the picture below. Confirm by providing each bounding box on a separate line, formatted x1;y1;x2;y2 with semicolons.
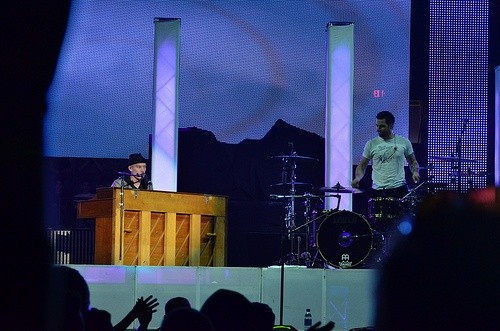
127;153;148;166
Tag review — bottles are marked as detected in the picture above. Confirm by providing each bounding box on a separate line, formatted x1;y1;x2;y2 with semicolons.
304;309;312;331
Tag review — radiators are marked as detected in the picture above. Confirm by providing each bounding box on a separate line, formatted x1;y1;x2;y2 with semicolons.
48;227;95;264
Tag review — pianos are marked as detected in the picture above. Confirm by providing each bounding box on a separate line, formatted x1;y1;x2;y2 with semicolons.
75;184;227;266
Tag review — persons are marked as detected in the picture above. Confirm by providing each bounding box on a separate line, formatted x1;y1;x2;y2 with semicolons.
373;189;500;331
352;111;420;244
111;153;153;190
54;265;334;331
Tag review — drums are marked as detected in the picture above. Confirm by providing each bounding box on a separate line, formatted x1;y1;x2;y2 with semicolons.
313;208;374;267
366;195;408;225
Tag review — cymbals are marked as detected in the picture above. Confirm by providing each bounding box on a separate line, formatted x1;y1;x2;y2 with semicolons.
429;156;479;162
274;181;311;185
317;188;365;195
270;154;319;161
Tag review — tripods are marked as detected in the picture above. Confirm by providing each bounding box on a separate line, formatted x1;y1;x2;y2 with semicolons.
277;159;305;266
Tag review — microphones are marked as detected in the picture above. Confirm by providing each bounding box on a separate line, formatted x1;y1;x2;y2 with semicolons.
342;236;350;241
142;173;152;185
